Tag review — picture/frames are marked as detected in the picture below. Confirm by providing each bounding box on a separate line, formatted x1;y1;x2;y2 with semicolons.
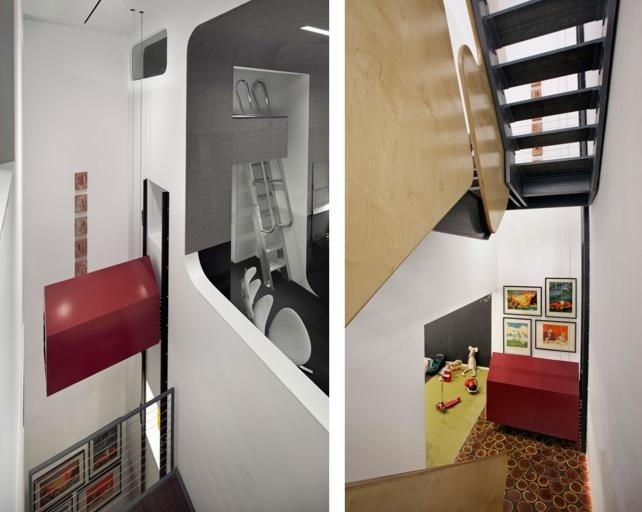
503;278;577;359
33;416;122;511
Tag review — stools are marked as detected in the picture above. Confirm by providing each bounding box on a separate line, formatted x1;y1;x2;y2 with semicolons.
486;352;581;442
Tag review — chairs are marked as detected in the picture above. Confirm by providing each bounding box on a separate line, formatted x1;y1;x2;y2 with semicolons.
239;265;313;374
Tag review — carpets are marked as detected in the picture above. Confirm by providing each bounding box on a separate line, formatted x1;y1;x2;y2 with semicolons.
453;405;591;511
